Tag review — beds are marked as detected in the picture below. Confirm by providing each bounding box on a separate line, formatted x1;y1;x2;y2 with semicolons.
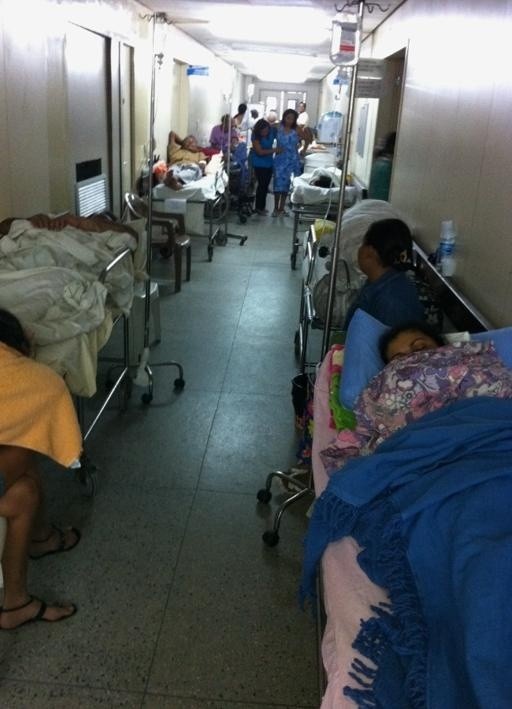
308;327;510;708
288;142;429;369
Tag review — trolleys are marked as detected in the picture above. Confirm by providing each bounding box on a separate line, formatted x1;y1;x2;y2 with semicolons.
0;213;137;495
288;172;367;270
293;225;434;371
205;192;229;222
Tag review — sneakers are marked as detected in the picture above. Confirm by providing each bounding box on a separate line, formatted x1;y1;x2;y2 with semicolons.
254;207;291;217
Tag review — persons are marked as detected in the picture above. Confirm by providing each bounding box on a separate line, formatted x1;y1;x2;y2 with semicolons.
0;211;139;242
314;176;335;188
210;102;312;217
380;322;442;363
164;131;206;189
368;132;397;201
292;219;418;416
1;308;81;631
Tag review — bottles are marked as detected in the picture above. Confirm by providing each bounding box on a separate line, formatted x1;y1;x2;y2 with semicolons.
329;12;360;79
438;220;458;278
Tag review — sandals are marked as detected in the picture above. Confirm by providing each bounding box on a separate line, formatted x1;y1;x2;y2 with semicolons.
30;525;81;559
1;594;76;628
